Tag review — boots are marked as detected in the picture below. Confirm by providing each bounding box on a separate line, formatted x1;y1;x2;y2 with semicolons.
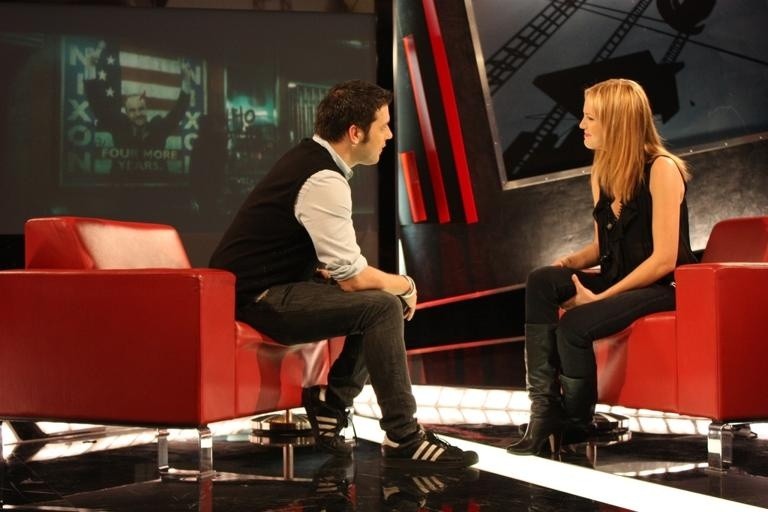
504;322;564;456
517;375;599;446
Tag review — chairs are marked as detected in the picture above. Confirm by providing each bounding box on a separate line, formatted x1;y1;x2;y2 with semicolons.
559;216;768;473
0;216;348;475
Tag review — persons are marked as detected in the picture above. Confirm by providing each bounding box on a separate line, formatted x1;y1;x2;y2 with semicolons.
507;77;694;455
86;64;191;181
208;80;478;471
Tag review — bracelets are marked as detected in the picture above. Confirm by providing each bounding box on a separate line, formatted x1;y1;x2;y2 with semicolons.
402;274;416;298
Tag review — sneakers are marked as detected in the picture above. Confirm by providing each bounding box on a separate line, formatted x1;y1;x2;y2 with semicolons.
301;382;358;459
380;429;480;473
